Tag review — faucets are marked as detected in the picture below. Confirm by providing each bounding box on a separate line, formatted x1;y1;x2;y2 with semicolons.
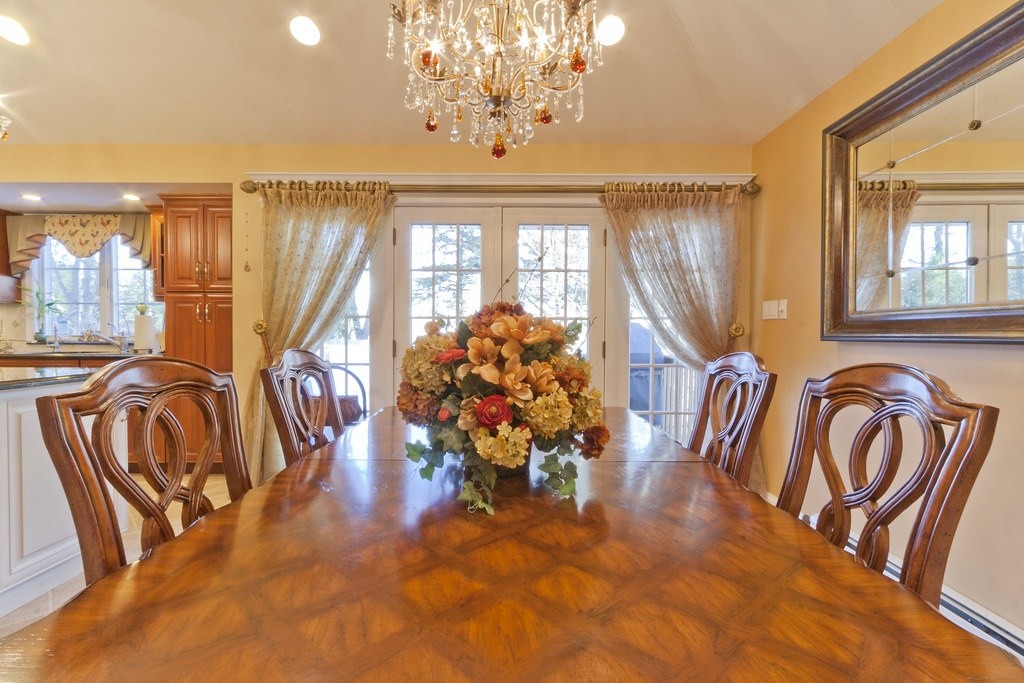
46;326;61;352
91;322;129;355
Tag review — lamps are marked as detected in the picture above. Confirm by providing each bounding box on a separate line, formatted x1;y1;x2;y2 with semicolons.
385;0;604;161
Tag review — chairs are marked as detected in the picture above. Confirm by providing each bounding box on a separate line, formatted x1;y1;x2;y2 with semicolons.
35;354;253;586
775;362;1002;612
260;349;346;467
687;352;778;489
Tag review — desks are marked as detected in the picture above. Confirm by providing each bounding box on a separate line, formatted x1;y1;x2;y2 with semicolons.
0;404;1024;683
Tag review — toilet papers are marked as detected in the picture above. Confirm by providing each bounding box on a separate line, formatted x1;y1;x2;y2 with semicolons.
134;315;160;350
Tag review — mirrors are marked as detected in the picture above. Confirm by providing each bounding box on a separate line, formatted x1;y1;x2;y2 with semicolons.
818;0;1024;349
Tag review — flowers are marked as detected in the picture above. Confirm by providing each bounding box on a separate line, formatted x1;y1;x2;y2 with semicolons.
394;246;608;513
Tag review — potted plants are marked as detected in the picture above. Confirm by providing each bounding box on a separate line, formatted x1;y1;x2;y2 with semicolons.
13;285;62;342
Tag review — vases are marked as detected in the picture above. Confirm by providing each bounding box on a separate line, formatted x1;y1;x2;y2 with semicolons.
462;440;531;476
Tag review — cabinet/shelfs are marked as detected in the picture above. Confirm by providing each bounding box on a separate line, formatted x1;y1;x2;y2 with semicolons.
2;358;163;472
146;204;167;302
164;292;233;462
163;194;234;292
302;364;367;449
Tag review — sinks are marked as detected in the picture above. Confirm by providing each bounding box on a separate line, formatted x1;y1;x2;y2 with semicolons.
27;350;112;354
